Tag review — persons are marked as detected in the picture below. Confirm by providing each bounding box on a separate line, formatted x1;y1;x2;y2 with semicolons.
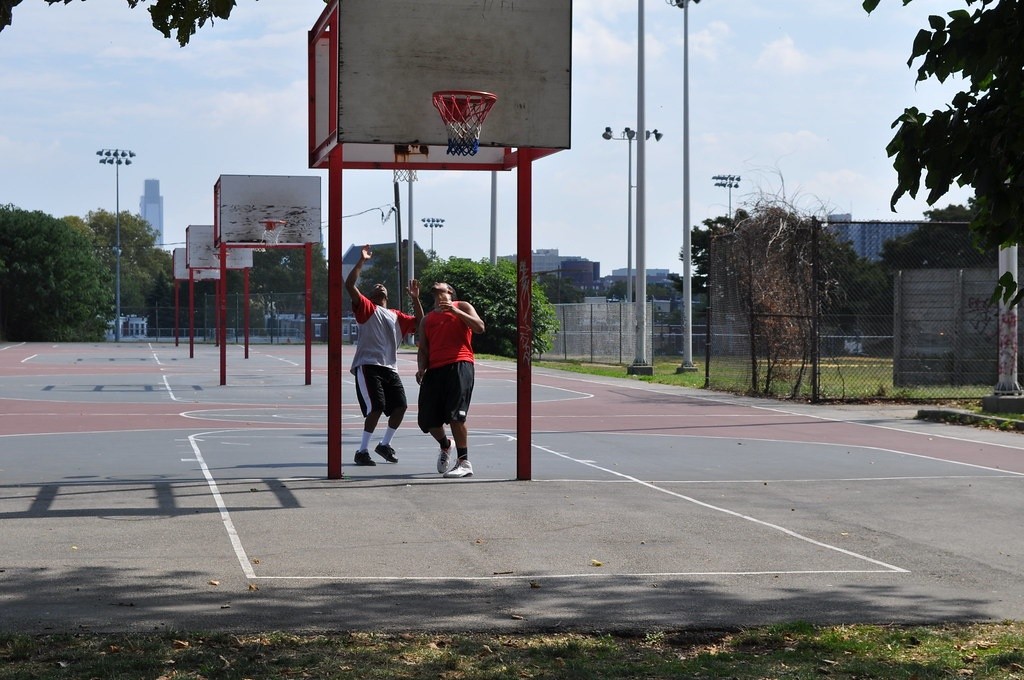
416;282;484;478
345;245;424;466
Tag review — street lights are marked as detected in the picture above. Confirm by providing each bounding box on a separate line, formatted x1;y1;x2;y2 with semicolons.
94;147;137;343
664;0;703;377
420;216;445;262
601;127;666;360
713;175;741;221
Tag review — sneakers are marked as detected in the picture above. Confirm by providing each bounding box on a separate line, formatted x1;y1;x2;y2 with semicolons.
437;438;455;474
442;459;474;478
354;450;376;466
375;441;398;462
